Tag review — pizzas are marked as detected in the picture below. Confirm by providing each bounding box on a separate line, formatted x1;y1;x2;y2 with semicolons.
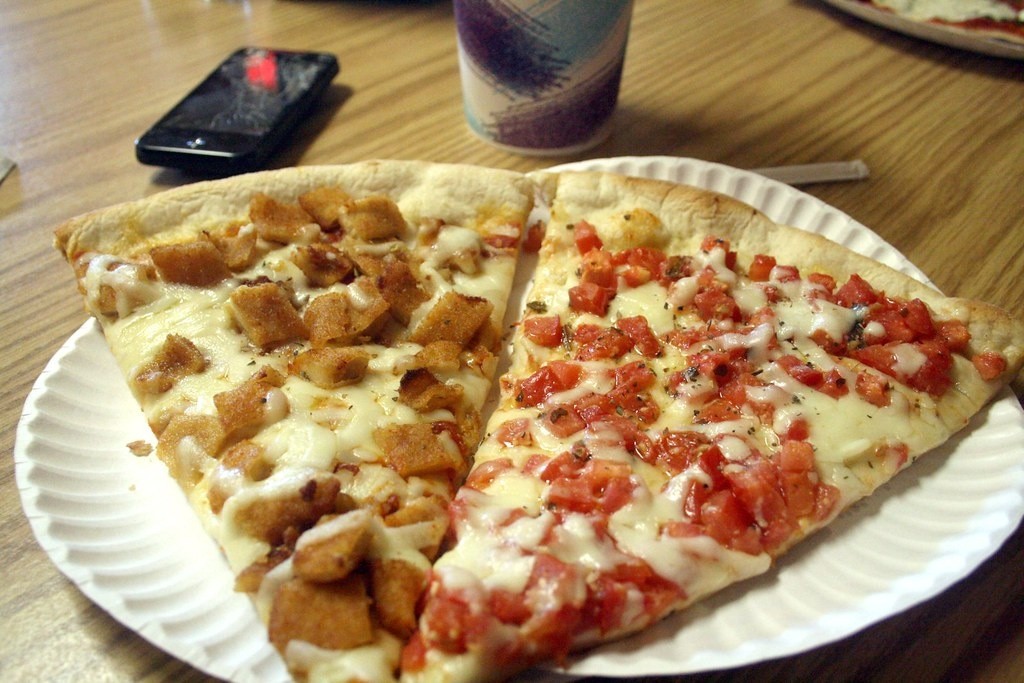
51;158;537;683
388;172;1024;683
860;0;1024;43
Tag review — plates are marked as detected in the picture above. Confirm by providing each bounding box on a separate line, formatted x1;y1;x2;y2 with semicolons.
13;157;1024;683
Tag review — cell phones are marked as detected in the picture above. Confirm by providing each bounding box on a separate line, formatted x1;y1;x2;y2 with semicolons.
135;47;337;175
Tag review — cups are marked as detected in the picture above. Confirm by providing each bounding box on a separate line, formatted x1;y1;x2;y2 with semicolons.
455;0;634;155
